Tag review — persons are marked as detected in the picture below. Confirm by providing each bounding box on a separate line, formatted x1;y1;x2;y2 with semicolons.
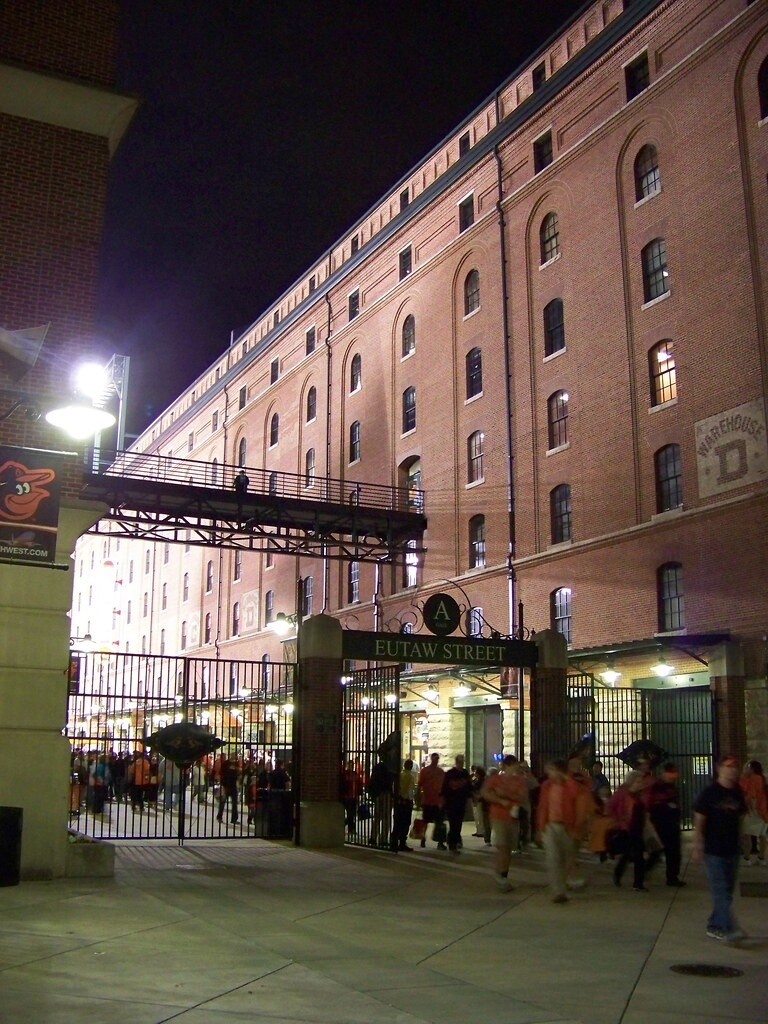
233;470;249;494
341;753;488;856
70;748;293;824
693;753;747;941
480;747;687;904
740;760;768;867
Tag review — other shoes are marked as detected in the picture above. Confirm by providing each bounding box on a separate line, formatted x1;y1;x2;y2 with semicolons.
437;843;447;850
493;873;512;892
612;874;622;888
389;842;398;853
379;842;390;846
705;925;746;940
476;834;484;837
666;876;688;887
420;839;426;848
511;848;521;854
633;882;648;892
398;844;413;852
367;838;377;846
552;895;567;904
750;854;767;865
450;849;460;855
472;832;478;836
737;854;752;866
521;849;529;855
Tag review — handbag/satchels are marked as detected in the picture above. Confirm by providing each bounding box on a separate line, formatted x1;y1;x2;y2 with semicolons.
432;824;447;841
409;805;426;840
357;795;371;820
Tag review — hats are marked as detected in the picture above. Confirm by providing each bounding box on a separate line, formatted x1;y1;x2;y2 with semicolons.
659;761;681;772
717;755;741;766
